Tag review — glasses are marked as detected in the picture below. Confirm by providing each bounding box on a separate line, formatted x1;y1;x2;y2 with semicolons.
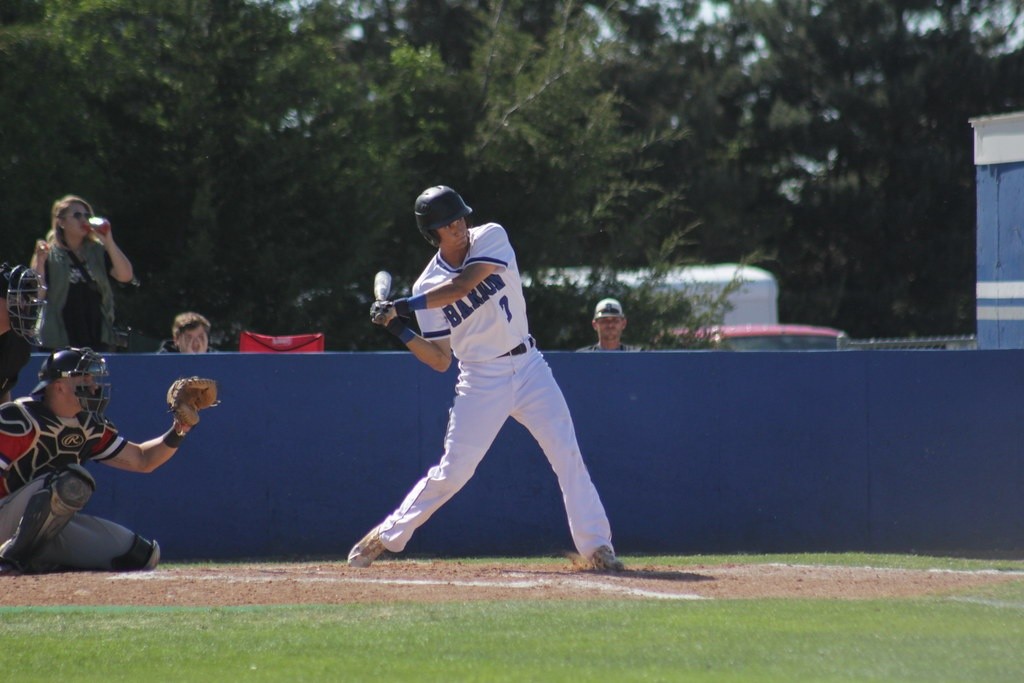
63;212;91;220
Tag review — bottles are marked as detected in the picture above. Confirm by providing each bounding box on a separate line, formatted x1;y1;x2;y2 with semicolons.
80;216;110;234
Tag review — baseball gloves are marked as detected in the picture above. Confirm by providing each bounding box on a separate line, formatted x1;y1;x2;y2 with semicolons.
164;374;223;436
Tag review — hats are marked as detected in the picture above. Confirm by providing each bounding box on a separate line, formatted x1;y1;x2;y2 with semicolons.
593;298;625;321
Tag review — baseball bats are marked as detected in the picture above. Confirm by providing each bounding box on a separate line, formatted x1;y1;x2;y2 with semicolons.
372;270;392;324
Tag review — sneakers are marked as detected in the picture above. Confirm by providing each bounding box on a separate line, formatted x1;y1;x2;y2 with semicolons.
589;546;625;573
346;524;394;569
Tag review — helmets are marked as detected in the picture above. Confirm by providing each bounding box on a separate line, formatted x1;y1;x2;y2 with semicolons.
413;185;473;247
0;263;31;298
31;348;95;394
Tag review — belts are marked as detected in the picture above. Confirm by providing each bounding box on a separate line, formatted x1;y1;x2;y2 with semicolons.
501;337;538;358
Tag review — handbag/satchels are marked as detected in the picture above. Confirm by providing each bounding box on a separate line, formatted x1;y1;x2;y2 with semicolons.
105;324;130;350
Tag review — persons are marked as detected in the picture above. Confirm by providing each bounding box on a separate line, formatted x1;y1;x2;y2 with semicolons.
0;346;217;573
347;184;624;570
30;194;134;353
158;312;212;353
579;297;642;351
0;263;47;405
